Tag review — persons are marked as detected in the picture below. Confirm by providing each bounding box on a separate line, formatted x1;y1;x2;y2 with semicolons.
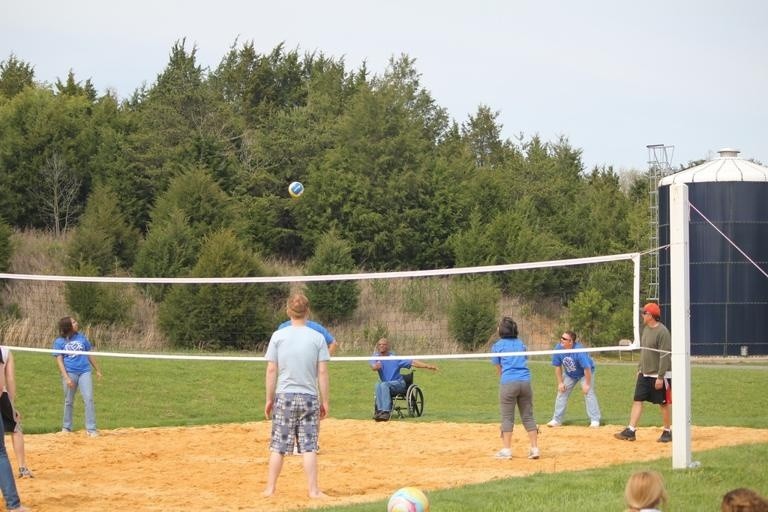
489;316;541;462
0;351;32;512
612;301;673;443
544;329;602;429
367;335;439;423
258;293;334;503
274;319;333;456
49;316;103;439
0;343;34;481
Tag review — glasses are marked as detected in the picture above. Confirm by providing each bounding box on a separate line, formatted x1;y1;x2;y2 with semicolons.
644;312;650;315
561;336;571;341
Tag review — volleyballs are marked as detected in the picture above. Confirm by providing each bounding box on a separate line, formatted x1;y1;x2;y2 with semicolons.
387;487;429;512
289;182;304;197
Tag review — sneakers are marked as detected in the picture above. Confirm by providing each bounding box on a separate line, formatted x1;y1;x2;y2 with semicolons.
547;419;562;427
61;428;70;432
372;412;389;420
17;467;35;479
527;448;540;459
87;430;96;437
590;420;600;427
494;448;514;459
614;427;635;441
657;430;672;442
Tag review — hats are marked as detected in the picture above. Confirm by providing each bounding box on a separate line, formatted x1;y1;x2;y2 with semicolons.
639;302;660;317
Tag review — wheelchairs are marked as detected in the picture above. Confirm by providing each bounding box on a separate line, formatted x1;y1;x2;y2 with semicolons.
371;368;425;420
